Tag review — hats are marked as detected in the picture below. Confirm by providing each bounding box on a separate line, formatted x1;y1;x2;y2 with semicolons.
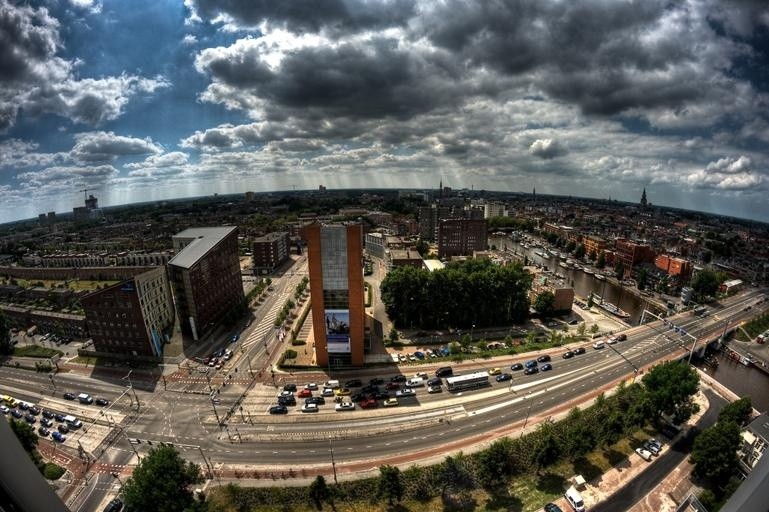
0;394;81;443
63;392;109;406
203;334;242;369
636;438;662;462
42;333;72;344
565;488;585;512
544;502;564;512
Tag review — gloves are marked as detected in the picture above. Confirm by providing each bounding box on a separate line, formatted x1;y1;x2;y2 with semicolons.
494;231;605;281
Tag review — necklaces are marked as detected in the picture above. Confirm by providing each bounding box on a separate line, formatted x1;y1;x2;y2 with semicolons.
589;275;632;321
